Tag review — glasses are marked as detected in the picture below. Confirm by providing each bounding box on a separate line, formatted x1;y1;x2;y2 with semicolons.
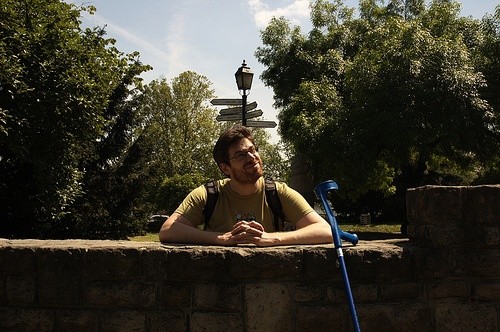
229;147;256;160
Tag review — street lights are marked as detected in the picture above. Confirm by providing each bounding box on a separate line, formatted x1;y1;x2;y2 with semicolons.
234;59;254;127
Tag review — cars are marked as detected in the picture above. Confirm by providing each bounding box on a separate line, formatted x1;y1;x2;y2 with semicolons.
145;214;170;233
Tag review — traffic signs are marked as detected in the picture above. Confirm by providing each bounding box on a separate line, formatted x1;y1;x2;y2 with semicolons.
219;107;242;115
244;101;258;112
245;109;264;120
210;99;243;105
246;120;278;128
216;114;242;122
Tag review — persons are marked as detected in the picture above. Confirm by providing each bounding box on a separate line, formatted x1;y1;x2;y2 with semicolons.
158;124;334;247
